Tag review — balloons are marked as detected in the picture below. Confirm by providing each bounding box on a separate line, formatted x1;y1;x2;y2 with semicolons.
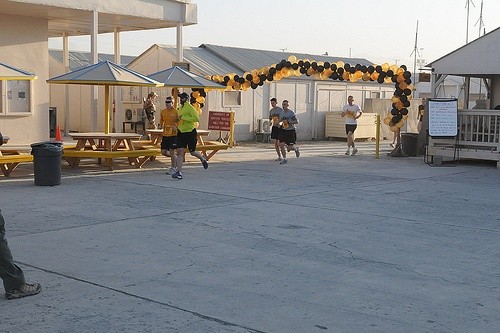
190;88;210;128
205;55;415;133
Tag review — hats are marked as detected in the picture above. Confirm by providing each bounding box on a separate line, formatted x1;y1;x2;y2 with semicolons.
178;93;189;98
148;92;158;97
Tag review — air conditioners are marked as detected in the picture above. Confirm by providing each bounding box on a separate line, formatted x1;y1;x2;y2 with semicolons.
125;107;143;122
257;119;271;133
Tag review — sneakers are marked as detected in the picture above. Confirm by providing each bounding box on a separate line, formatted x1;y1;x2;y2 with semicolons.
172;171;183;179
166;168;177;175
5;281;42;299
201;156;208;169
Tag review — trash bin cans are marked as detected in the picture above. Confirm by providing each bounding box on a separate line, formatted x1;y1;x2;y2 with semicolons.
401;133;419;155
31;140;62;185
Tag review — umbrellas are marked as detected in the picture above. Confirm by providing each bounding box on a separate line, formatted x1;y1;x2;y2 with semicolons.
147;65;226;161
0;62;37;81
46;59;164;167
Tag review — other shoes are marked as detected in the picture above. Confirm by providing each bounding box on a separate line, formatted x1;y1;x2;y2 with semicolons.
295;147;300;157
275;158;282;161
286;145;290;152
280;159;287;164
345;150;350;155
350;149;358;156
390;143;395;148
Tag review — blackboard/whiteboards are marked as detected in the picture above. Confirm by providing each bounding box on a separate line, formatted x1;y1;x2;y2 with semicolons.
428;98;459;139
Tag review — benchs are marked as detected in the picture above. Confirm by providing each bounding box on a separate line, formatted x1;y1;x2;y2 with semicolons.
63;140;228;167
0;155;34;177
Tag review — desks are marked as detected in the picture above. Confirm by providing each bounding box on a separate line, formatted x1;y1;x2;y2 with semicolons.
67;132;143;169
0;145;33;177
145;128;211;161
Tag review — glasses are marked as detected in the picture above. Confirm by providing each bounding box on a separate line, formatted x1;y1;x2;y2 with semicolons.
165;101;172;103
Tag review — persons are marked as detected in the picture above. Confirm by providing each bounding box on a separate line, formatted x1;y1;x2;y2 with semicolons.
276;100;300;164
157;96;181;175
172;92;208;178
390;131;401;148
341;95;363;156
0;132;42;300
417;98;426;132
269;98;290;161
